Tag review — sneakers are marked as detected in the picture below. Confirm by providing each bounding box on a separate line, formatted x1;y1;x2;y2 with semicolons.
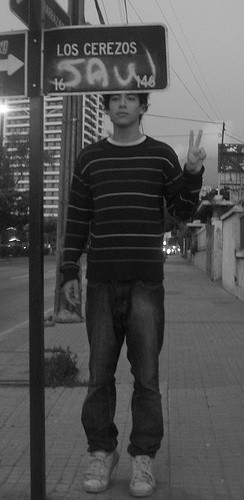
83;450;118;492
129;455;157;496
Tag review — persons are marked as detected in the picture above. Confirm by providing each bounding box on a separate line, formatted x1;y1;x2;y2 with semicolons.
60;92;207;496
